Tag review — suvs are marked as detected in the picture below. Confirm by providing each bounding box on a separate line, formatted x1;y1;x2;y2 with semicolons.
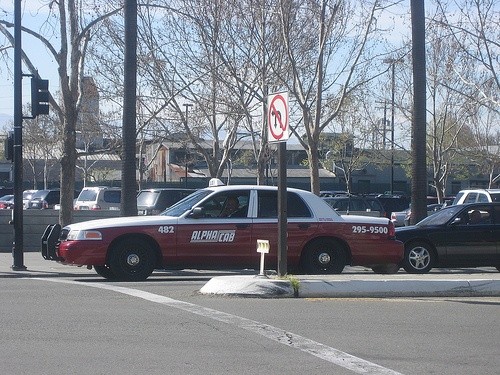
135;188;222;217
74;186;121;210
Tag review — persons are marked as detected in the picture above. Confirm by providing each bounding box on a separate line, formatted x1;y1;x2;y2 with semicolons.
470;211;484;224
226;198;242;217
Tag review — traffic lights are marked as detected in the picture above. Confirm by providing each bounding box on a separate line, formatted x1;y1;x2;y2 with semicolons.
4;138;13;160
31;77;50;115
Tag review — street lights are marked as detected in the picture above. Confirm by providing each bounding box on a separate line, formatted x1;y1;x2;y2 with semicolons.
183;104;193;176
384;59;403;196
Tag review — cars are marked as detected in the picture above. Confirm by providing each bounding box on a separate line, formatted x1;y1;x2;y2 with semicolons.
319;187;500;227
394;203;500;274
40;176;404;286
0;186;79;211
